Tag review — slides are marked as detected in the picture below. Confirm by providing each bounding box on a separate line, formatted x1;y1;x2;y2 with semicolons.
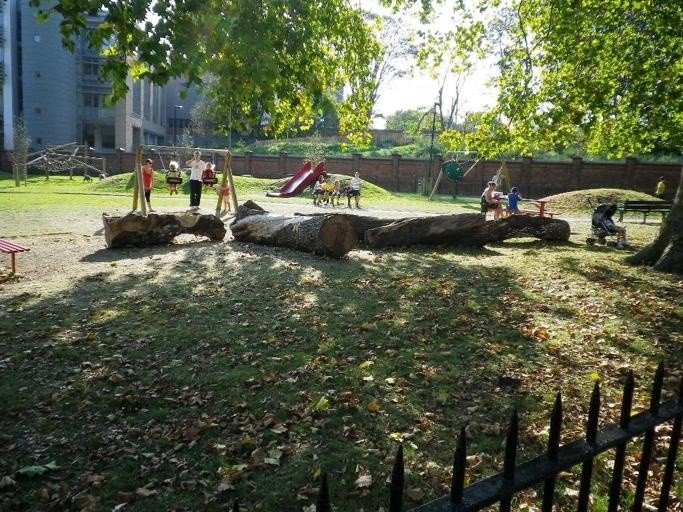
279;159;324;196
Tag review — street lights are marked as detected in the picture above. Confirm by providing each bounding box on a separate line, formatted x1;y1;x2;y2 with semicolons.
174;104;183;145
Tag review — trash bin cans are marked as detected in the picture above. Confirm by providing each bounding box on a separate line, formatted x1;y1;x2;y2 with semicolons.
418;178;433;196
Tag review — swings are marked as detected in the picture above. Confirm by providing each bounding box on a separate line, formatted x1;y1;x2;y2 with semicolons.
441;151;485;181
199;147;218;184
157;146;184;184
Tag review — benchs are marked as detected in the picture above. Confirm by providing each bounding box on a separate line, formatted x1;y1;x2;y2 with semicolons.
502;207;561;218
0;239;30;273
617;200;673;224
312;183;358;205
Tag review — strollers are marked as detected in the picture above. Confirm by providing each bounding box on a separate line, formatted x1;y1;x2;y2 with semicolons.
586;196;624;249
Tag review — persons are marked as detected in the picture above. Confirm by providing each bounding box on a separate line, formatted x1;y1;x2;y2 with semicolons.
321;178;331;202
140;158;155;210
601;214;628;249
330;180;341;208
185;151;205;210
164;160;180;195
343;180;352;195
347;171;360;209
312;174;325;205
222;180;230;212
201;162;214;188
505;186;522;212
479;182;500;220
655;175;665;199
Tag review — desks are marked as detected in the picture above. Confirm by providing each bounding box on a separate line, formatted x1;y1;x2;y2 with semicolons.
494;196;557;217
